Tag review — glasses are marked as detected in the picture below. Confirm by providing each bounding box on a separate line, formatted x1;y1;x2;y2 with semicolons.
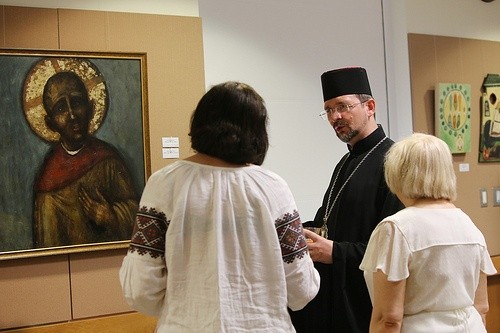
319;103;363;118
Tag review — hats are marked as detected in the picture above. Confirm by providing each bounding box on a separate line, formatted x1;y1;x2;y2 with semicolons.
321;66;372;102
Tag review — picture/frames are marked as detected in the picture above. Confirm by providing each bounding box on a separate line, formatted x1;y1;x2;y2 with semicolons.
0;49;152;261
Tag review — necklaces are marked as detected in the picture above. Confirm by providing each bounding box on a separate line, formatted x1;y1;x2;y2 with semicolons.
320;135;389;239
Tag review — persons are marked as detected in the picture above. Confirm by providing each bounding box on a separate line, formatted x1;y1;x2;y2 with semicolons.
359;133;497;332
117;81;320;332
296;67;404;333
